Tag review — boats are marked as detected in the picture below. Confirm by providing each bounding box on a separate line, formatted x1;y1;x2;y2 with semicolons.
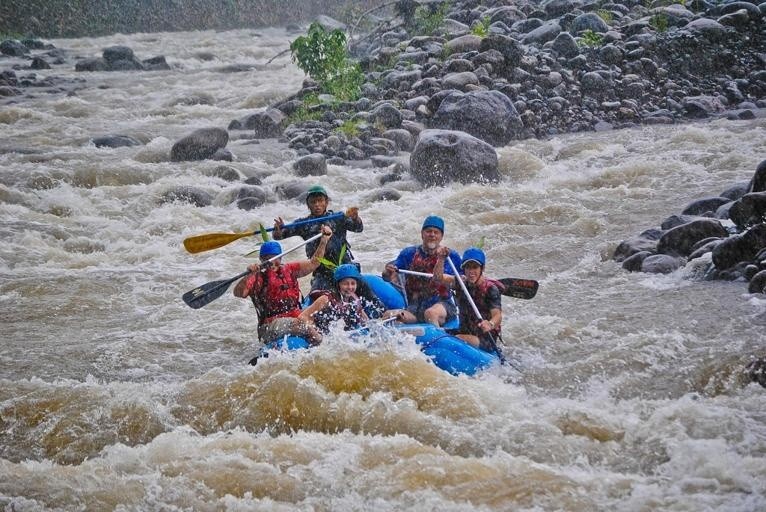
262;274;499;381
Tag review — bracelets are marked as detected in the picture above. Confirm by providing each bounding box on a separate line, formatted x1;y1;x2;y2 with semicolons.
489;321;496;329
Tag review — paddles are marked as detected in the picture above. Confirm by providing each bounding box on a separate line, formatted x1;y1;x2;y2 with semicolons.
396;267;538;299
184;207;356;255
183;226;330;310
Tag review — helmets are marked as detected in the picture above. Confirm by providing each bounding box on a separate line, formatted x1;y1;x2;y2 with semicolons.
307;185;327;196
334;263;361;283
462;247;486;270
260;242;282;258
423;216;445;230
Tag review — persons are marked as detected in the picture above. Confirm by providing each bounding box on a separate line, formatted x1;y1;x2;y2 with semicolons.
432;246;503;351
271;184;386;311
381;215;463;329
294;263;408;344
232;223;333;346
